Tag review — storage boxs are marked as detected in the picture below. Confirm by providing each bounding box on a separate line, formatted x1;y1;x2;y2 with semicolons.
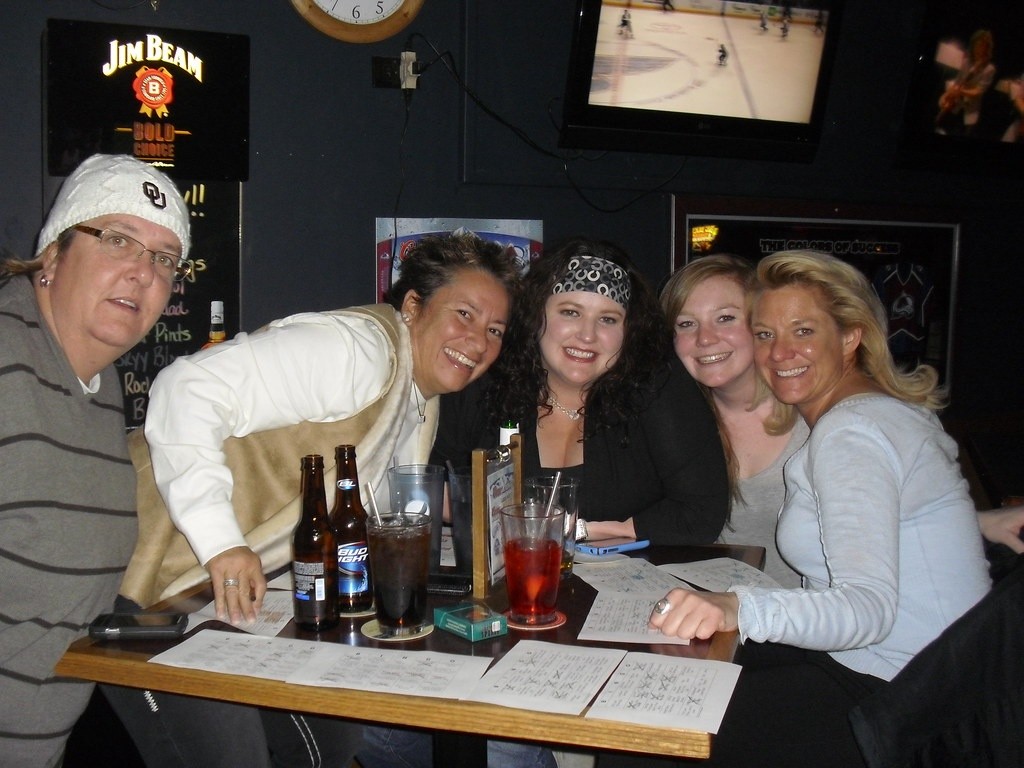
434;599;507;642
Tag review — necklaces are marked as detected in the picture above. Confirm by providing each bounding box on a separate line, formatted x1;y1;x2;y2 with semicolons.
544;393;583;420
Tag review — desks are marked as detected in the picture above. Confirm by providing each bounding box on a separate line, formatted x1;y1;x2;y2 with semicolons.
53;538;766;768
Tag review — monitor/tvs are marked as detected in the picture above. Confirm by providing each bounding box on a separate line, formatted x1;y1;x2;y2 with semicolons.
557;0;844;166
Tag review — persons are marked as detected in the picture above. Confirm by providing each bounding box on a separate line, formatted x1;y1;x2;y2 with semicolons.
424;237;748;768
718;44;728;66
650;251;1024;768
124;242;526;768
760;10;769;31
932;31;1000;138
0;153;191;768
660;255;1024;588
779;6;824;39
660;0;675;13
621;8;632;33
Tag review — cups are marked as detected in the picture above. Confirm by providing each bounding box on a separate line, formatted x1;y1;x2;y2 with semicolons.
365;512;432;637
387;464;446;588
522;476;581;580
445;455;473;579
499;502;567;626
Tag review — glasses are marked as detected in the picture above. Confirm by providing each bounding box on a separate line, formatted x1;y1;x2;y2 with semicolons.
73;224;192;281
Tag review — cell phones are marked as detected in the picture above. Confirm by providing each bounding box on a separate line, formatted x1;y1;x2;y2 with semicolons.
573;537;650;556
426;572;473;595
90;611;189;638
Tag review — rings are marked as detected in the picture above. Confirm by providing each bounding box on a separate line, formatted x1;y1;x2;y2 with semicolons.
654;597;671;614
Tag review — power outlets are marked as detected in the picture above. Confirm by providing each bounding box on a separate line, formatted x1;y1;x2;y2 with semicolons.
371;55;421;90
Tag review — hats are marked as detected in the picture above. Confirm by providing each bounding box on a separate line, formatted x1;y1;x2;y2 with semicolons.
35;153;191;277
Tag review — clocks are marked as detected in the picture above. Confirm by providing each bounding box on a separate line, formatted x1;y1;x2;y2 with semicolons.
290;0;424;44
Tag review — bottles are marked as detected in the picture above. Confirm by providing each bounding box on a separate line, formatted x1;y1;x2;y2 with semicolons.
291;454;340;633
498;418;520;447
328;444;374;614
198;299;227;350
